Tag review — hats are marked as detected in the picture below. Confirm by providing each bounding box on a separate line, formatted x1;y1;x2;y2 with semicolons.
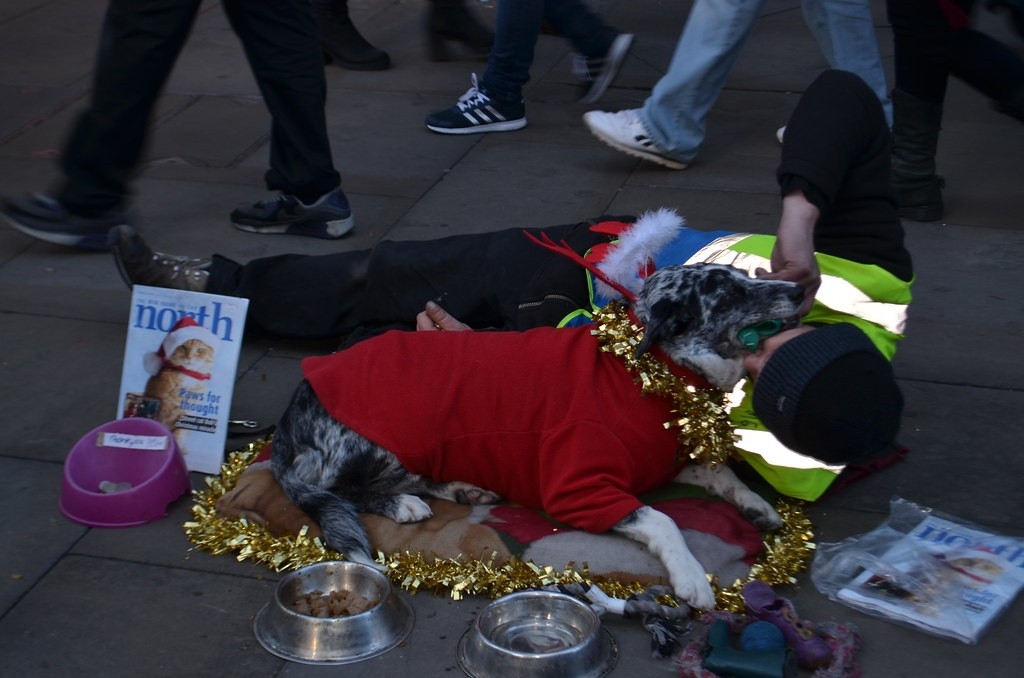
751;321;906;464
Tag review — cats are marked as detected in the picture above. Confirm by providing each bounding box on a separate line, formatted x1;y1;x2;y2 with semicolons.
905;547;1003;617
143;316;220;470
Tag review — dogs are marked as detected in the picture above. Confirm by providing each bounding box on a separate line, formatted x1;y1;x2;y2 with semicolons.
270;262;806;612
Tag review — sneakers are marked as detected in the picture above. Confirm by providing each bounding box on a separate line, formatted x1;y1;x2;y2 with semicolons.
108;224;213;292
230;186;357;238
582;109;688;169
572;24;635;105
776;126;786;141
425;73;527;134
0;191;136;252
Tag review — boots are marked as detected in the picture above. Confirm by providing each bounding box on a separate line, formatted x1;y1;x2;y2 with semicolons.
426;0;494;60
995;43;1024;123
891;88;944;221
319;0;391;68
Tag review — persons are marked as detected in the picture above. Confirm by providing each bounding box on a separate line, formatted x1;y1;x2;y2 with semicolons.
109;69;914;505
315;1;494;68
0;0;357;251
886;1;1023;222
426;0;634;135
586;0;893;169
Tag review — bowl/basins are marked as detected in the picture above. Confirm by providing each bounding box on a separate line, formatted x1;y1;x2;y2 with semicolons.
455;591;619;678
58;417;192;527
253;560;415;666
